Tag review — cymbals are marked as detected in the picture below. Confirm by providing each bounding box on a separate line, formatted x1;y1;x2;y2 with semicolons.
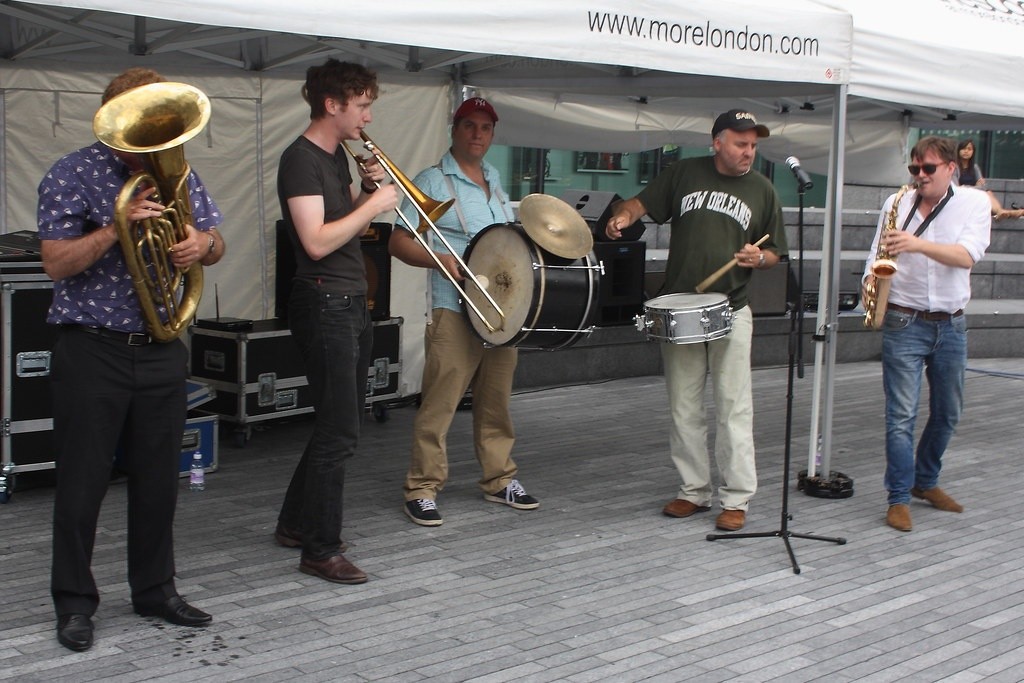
520;194;593;260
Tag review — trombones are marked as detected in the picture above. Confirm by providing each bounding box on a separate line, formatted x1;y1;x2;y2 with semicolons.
301;79;506;335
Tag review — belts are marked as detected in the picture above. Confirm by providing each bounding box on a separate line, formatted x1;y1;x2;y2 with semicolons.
64;323;157;346
887;303;965;320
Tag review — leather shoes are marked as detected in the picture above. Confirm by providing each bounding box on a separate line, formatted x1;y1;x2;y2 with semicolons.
57;614;94;651
275;519;348;553
143;595;213;626
299;551;368;585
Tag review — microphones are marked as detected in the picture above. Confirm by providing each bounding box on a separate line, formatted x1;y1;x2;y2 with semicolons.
786;156;814;190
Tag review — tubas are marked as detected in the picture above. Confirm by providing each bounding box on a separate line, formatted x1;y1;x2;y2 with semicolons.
93;80;203;342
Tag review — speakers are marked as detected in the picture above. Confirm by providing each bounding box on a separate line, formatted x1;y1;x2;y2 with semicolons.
277;219;392;322
557;188;646;327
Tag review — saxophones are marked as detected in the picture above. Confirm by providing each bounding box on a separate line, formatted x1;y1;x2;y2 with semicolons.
864;183;918;329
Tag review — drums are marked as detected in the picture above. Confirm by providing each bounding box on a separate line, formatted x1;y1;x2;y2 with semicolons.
458;222;600;351
634;290;736;344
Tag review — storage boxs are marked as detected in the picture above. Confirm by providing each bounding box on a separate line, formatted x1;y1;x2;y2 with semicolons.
0;262;403;503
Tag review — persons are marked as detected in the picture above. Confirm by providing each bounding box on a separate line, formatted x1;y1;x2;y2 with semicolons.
38;69;225;652
390;96;541;527
950;138;1024;223
276;60;399;586
861;136;993;533
604;109;790;532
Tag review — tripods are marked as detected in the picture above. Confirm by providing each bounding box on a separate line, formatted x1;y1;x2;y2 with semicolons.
707;187;848;574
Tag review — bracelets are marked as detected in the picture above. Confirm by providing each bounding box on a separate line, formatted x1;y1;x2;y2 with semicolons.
758;251;766;268
361;180;380;194
206;231;215;254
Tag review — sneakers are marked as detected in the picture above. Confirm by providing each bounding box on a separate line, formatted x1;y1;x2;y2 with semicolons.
663;498;711;518
716;508;746;531
912;486;963;513
404;499;444;526
887;504;912;531
484;480;540;509
